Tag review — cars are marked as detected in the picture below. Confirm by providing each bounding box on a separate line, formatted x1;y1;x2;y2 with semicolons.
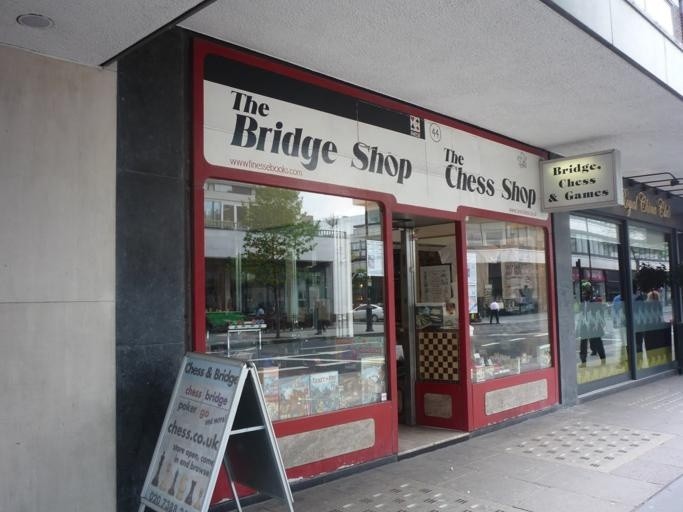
353;303;384;322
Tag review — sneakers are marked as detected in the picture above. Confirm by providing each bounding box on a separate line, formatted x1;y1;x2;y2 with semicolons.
590;351;597;355
600;356;606;365
578;362;586;368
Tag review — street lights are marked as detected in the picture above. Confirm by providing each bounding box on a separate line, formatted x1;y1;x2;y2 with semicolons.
244;253;248;314
366;278;374;332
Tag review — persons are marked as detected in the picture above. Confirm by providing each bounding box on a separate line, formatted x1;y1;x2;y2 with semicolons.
488;299;498;324
255;302;265;322
575;284;665;371
